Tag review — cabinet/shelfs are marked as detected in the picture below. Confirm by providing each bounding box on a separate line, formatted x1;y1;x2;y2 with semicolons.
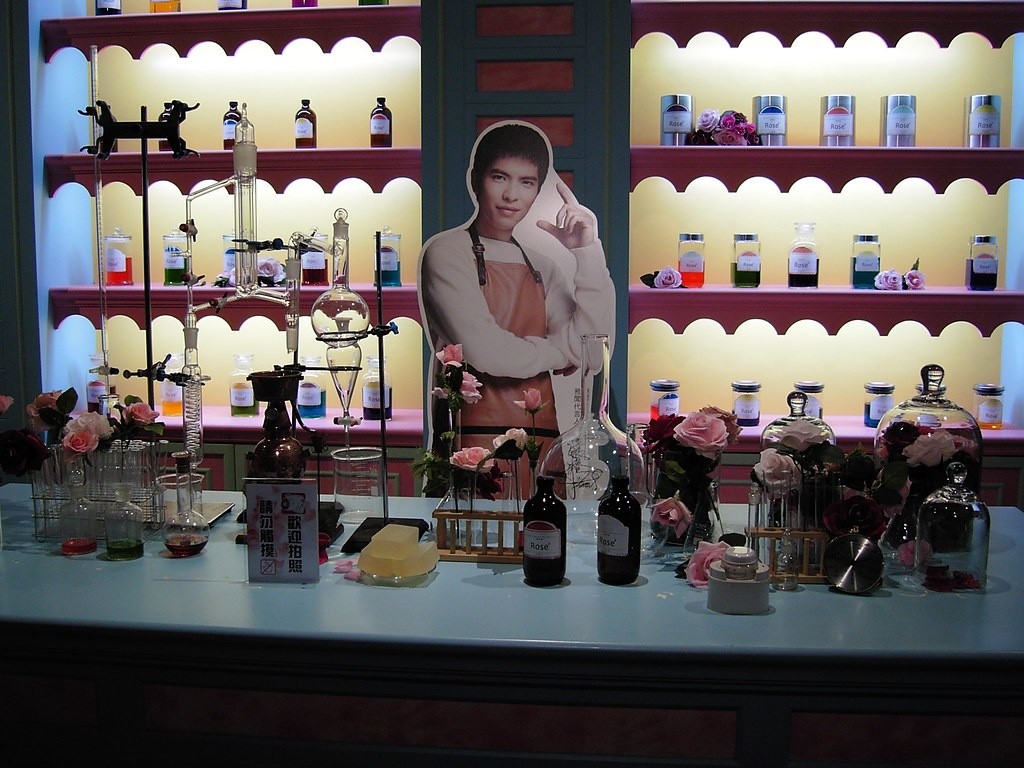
39;4;424;498
627;0;1024;508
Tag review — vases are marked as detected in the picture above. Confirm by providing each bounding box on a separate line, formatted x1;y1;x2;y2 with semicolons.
447;469;464;546
649;452;722;547
757;415;837;570
42;444;130;538
511;458;523;545
527;459;540;499
465;478;478;553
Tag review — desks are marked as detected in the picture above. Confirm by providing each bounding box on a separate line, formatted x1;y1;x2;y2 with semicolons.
0;482;1024;768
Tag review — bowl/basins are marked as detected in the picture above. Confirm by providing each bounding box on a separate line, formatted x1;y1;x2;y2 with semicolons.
356;563;438;589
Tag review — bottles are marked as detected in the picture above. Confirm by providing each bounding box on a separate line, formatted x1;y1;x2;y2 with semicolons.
652;223;1005;592
533;334;651;545
84;98;403;424
102;484;145;562
523;476;566;585
59;469;100;555
162;452;209;558
591;422;669;558
597;475;640;584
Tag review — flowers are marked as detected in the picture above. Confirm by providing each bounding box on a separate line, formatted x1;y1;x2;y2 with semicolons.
874;400;984;552
211;256;293;288
751;420;879;537
870;420;980;546
429;343;485;536
640;265;688;289
513;387;552;495
674;539;732;592
683;108;763;146
410;437;523;511
640;403;743;538
902;255;926;290
493;427;528;514
873;268;904;291
897;539;933;566
0;387;166;486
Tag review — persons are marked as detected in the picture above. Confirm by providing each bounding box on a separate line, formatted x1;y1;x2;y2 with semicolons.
421;125;618;499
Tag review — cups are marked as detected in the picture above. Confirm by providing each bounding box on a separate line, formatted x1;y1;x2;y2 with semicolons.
331;446;387;524
154;474;204;541
